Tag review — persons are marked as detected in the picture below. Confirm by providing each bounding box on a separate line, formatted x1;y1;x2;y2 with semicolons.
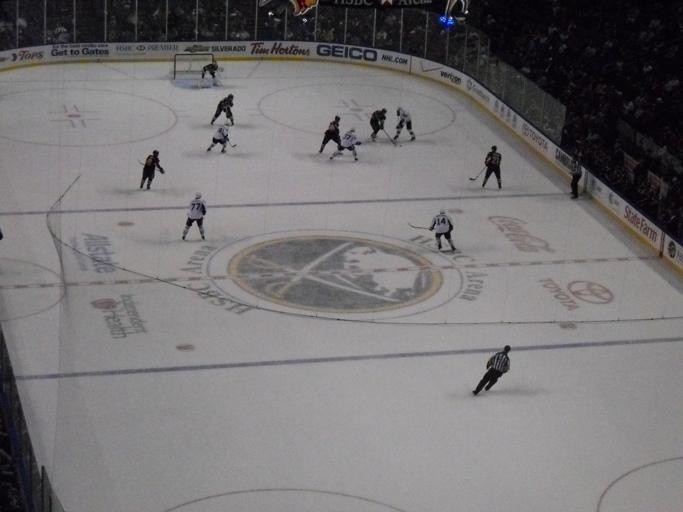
473;345;511;396
0;399;23;510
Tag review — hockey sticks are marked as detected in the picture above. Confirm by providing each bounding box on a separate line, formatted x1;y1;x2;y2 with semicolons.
382;128;402;147
137;159;160;172
469;166;487;180
229;141;237;147
407;223;428;229
